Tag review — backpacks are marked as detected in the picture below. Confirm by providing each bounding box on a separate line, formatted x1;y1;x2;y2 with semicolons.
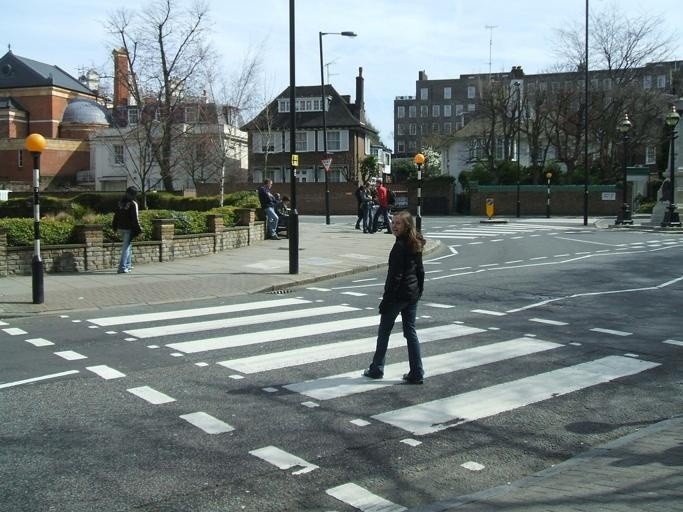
386;187;396;207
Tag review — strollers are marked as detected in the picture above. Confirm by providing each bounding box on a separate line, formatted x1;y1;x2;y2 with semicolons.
363;201;395;233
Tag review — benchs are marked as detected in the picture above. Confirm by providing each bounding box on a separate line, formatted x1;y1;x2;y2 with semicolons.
278;226;289;240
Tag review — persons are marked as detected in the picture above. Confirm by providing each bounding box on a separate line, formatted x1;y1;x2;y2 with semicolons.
258;178;291;240
355;177;392;234
112;186;144;273
363;210;426;384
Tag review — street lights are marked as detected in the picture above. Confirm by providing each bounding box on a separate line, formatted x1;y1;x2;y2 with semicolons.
315;28;360;227
512;80;525;221
414;151;426;257
545;170;552;223
21;130;50;307
614;111;636;227
660;105;683;229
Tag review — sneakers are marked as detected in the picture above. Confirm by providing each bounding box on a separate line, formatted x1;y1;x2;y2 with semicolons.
384;231;392;234
403;373;424;385
363;367;383;379
